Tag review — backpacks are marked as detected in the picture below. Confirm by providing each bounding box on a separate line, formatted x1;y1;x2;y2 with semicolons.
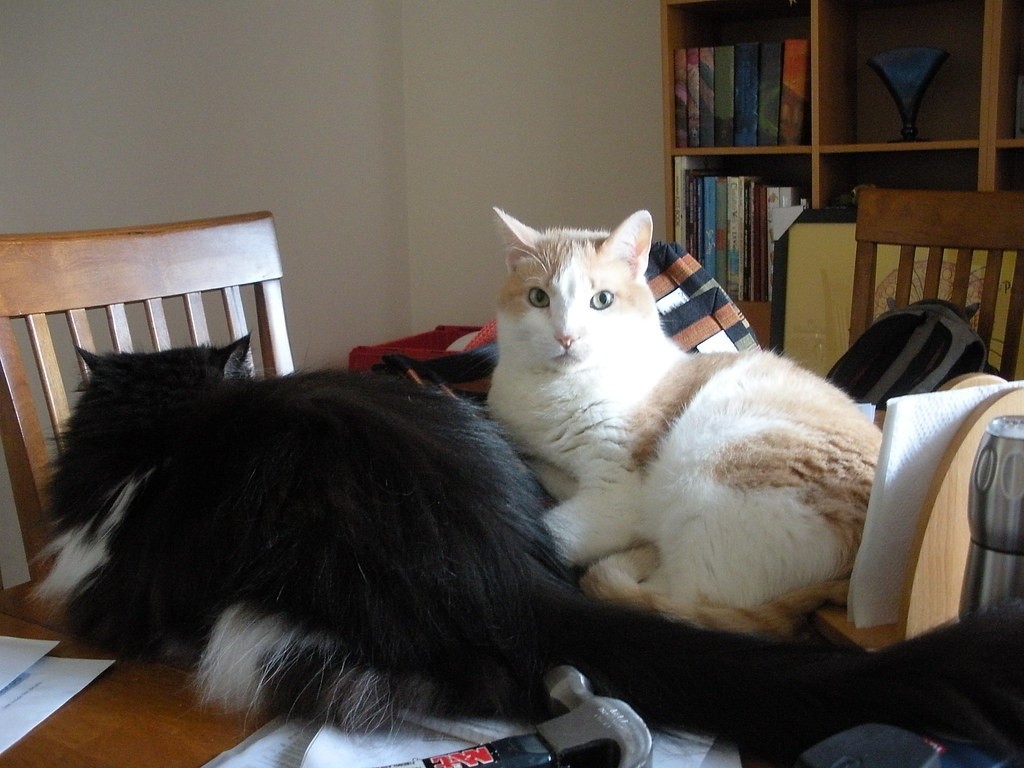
826;296;989;410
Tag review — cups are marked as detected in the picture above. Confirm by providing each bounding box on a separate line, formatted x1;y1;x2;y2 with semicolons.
956;415;1024;622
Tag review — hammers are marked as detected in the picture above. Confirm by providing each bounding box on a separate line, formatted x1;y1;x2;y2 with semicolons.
377;664;654;768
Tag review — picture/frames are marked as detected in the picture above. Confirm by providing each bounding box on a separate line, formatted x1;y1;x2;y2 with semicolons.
770;209;1024;383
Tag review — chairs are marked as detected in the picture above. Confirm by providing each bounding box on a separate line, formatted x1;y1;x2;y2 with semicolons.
850;187;1024;384
0;211;295;578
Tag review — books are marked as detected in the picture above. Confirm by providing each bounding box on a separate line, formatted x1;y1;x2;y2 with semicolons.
674;156;810;301
674;39;810;147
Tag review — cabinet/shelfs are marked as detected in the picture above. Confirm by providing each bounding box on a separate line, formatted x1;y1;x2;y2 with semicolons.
658;0;1024;352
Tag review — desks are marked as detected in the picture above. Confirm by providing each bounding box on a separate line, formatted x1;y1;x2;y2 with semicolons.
0;580;275;768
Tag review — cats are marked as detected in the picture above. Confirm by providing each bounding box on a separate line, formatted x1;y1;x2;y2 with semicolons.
46;329;1023;752
486;203;885;635
